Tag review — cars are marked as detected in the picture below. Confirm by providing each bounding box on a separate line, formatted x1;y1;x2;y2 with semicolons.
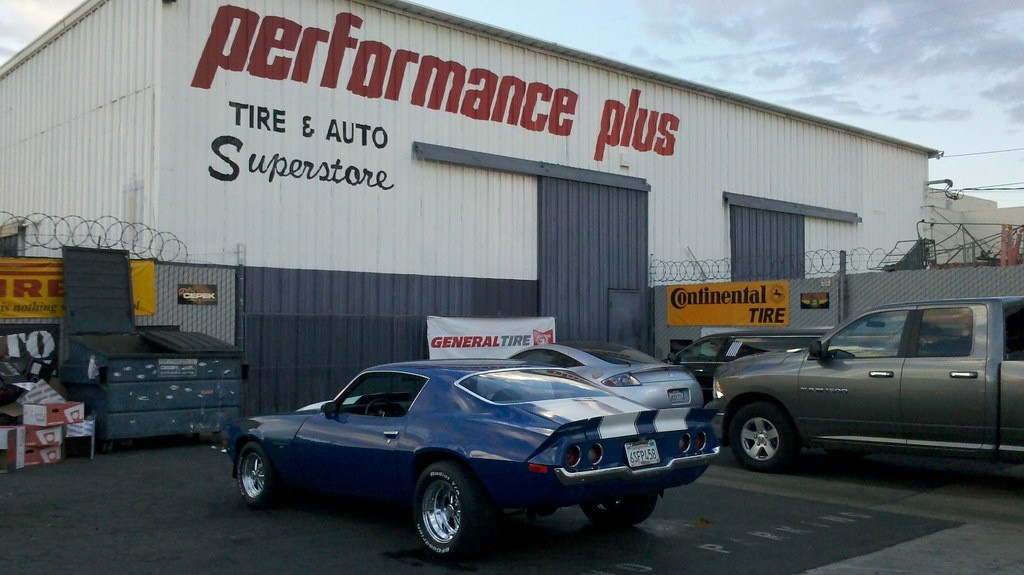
669;327;839;405
220;358;721;564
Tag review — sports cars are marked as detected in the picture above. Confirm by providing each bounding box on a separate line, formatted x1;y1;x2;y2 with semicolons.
508;340;704;415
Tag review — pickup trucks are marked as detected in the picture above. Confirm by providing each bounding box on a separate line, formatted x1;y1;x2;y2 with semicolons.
710;294;1021;473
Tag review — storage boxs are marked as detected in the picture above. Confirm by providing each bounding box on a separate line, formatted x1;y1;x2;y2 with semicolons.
0;378;95;475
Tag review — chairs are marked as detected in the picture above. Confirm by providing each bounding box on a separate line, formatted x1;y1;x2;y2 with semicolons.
491;390;522;403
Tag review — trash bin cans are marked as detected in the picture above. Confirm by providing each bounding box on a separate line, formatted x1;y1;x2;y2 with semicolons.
59;245;246;454
